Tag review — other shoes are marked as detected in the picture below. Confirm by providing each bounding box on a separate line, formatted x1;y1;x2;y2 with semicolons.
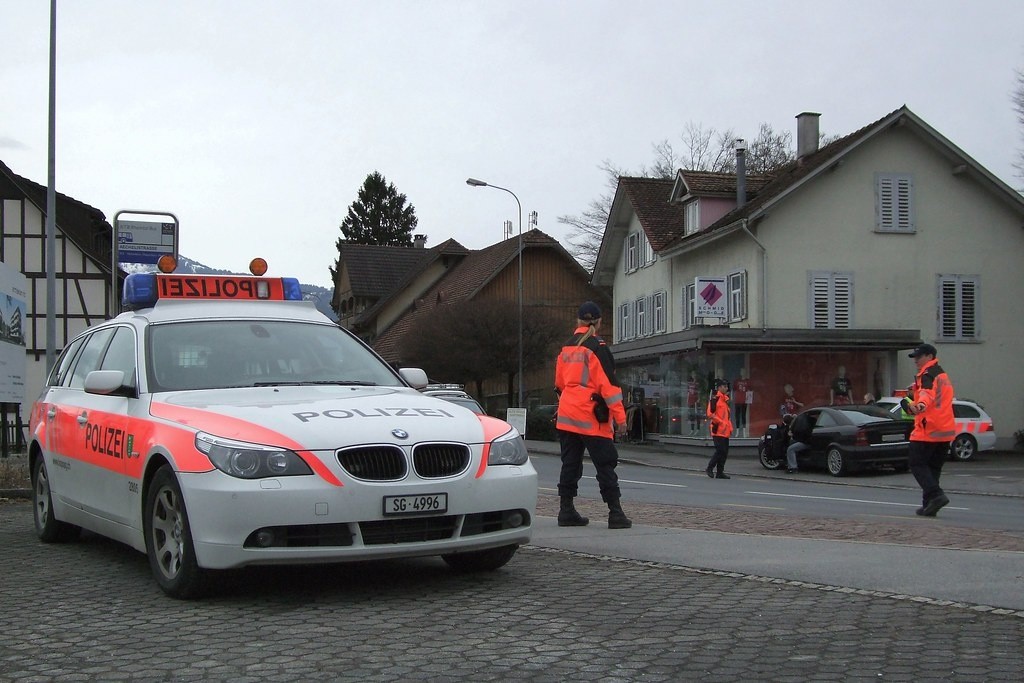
787;469;798;474
917;495;949;517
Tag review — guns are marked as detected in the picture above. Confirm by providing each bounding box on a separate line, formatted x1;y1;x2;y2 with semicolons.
590;393;609;423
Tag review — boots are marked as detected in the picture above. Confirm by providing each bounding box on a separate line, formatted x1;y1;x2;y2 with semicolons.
608;498;632;528
717;465;730;479
558;496;589;526
706;462;716;478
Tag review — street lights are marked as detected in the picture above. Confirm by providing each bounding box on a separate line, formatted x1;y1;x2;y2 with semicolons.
465;176;524;407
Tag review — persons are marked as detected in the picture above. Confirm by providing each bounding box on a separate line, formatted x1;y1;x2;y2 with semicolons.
689;370;702;439
830;367;854;408
700;379;733;479
905;344;957;516
782;384;804;414
734;381;747;439
556;301;633;529
782;414;814;474
863;393;876;409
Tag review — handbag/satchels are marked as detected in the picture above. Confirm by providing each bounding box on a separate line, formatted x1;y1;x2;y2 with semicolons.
745;390;753;404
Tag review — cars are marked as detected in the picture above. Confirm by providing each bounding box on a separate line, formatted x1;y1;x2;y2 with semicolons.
25;253;539;600
419;382;487;416
756;402;915;477
876;389;996;461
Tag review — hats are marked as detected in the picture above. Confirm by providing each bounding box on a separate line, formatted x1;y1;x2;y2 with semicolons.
909;344;937;358
717;380;730;388
906;383;916;389
579;301;601;320
